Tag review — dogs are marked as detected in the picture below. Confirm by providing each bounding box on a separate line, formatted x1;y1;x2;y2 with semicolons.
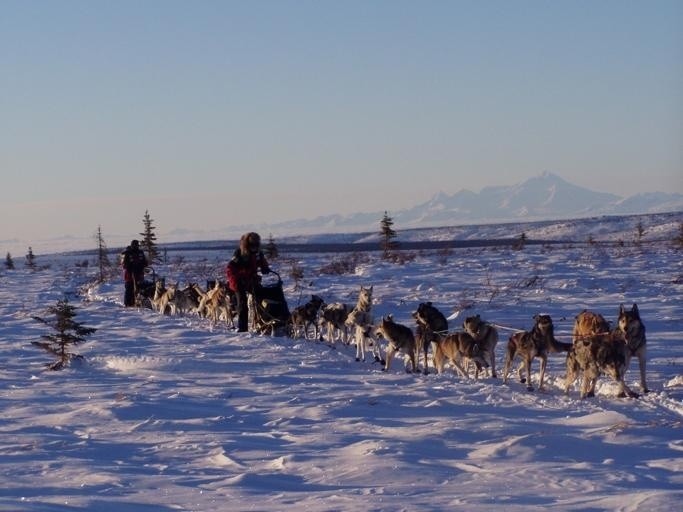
145;276;653;401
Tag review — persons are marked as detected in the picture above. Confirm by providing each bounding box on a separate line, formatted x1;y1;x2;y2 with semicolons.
226;232;270;332
120;240;148;306
